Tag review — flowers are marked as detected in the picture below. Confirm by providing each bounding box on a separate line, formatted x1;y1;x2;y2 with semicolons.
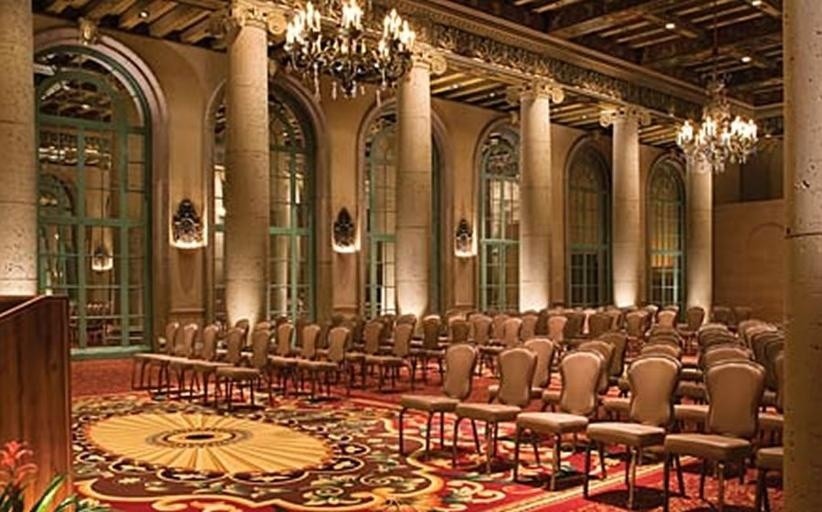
2;439;111;512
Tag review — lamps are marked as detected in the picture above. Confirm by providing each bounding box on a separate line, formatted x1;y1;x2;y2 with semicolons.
677;1;759;171
284;2;415;102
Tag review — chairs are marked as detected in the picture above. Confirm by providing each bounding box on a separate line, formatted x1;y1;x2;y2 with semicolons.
66;301;110;348
